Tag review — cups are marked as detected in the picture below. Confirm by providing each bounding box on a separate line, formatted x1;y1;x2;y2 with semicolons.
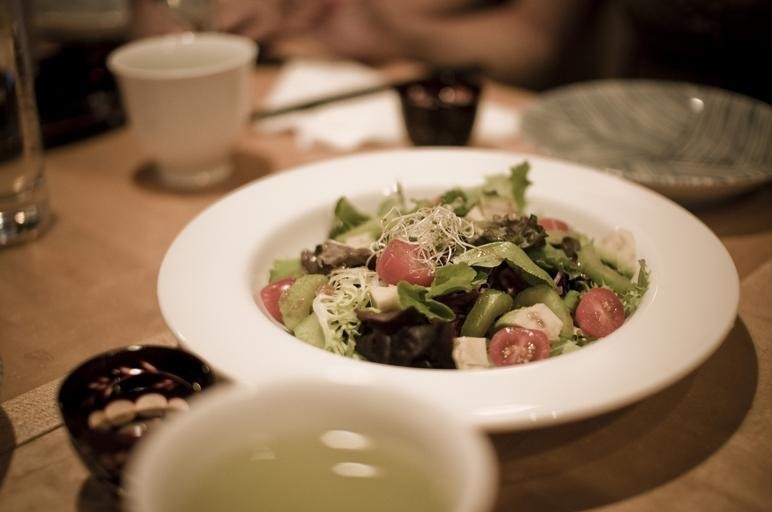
120;377;497;510
0;3;54;250
104;32;260;190
388;72;485;146
56;345;218;493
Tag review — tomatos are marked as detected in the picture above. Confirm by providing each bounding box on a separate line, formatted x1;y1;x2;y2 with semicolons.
576;288;625;338
489;328;550;367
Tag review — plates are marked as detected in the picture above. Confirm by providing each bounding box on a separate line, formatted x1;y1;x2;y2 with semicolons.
155;147;741;433
520;81;771;203
